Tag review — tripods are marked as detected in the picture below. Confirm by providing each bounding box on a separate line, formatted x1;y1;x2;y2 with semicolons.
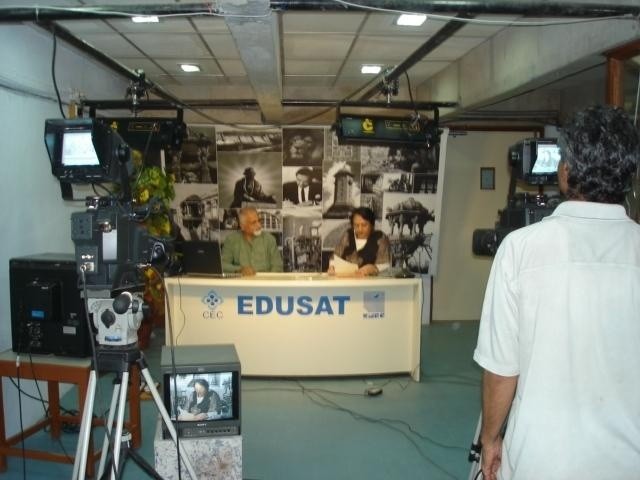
74;358;199;480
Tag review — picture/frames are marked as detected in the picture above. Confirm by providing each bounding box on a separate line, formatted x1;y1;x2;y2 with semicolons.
480;167;495;190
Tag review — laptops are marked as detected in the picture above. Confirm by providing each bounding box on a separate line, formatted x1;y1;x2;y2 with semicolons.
182;239;243;278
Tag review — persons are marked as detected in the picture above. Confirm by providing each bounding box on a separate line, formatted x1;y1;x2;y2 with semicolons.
220;207;284;277
469;98;639;479
229;168;275;209
283;168;323;205
327;206;392;279
177;378;223;422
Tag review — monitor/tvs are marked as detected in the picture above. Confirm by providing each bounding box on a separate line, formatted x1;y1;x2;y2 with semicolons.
160;344;241;439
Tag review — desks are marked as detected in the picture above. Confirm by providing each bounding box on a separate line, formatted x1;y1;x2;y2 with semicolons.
0;349;141;480
